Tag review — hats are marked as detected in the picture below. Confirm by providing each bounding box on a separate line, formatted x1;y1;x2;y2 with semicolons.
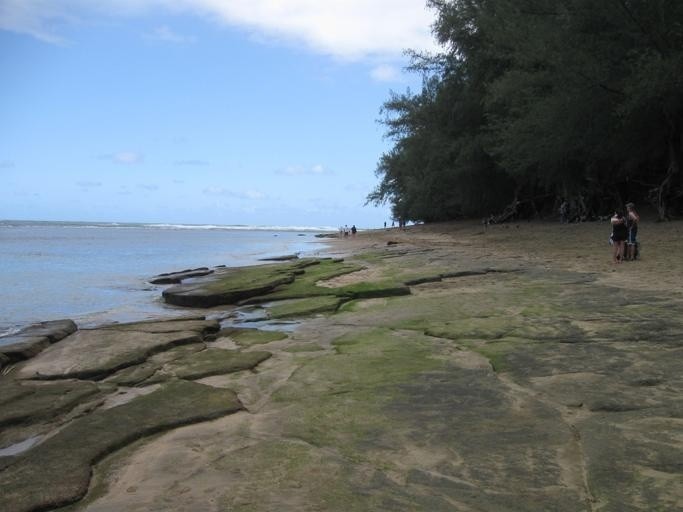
625;202;635;208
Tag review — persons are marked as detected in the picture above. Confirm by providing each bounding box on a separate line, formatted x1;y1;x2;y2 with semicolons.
609;208;627;264
559;200;569;223
350;225;356;240
624;202;640;261
338;224;349;239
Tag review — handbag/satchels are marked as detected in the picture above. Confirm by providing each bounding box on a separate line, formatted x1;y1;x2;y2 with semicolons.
609;233;616;247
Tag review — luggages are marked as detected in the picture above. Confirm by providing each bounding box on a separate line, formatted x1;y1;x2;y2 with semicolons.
624;228;638;260
